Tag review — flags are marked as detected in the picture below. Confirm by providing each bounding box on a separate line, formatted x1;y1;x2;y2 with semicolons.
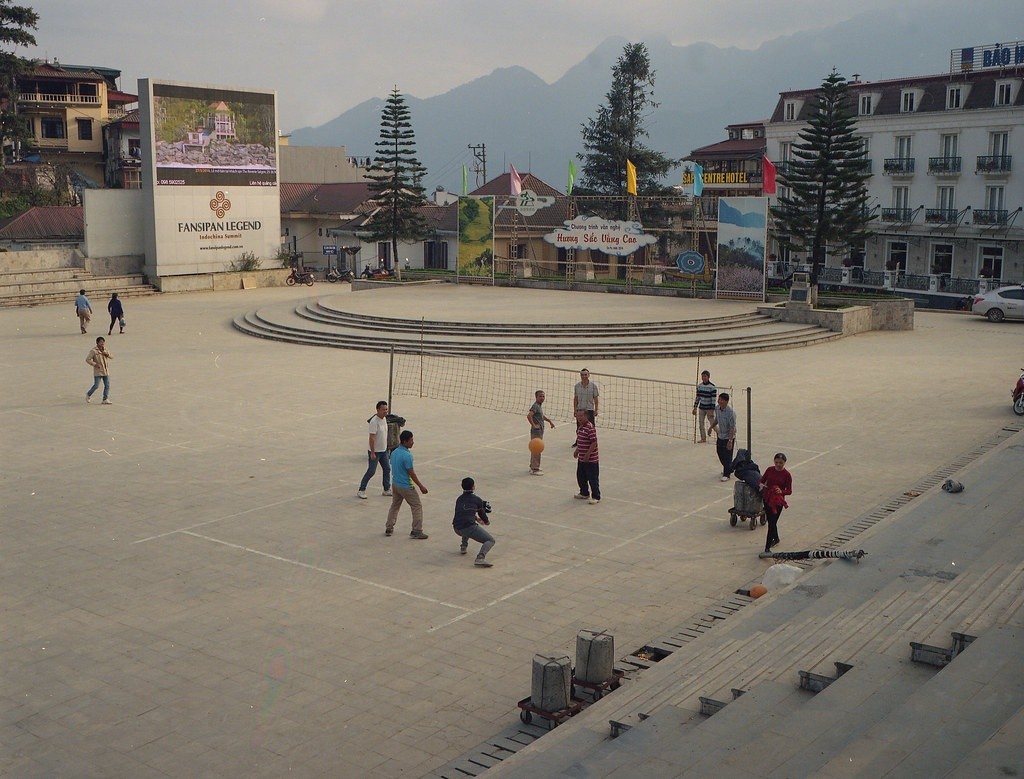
510;163;522;195
568;161;576;195
461;164;468;195
693;162;704;197
626;158;638;196
761;154;776;194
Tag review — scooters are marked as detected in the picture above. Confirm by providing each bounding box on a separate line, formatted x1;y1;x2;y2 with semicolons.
1010;368;1024;416
404;257;411;271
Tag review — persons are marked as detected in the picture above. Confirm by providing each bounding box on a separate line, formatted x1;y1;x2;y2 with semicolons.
107;293;126;335
385;431;428;539
709;393;738;482
86;337;114;404
451;477;495;566
573;409;602;504
527;391;556;476
571;368;599;447
757;453;793;552
356;401;393;499
74;290;93;333
692;371;720;443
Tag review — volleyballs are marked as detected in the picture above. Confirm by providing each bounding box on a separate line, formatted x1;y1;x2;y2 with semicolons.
529;438;544;454
750;586;767;598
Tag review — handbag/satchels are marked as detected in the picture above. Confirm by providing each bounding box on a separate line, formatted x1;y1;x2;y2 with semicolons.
119;317;127;328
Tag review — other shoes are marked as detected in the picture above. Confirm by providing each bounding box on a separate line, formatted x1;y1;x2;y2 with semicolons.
529;467;544;476
101;399;112;404
721;469;724;474
461;546;467;554
588;498;599;504
382;488;393;496
85;392;91;402
108;331;112;335
574;493;590;499
81;331;88;334
357;490;367;499
119;331;125;334
771;539;781;546
81;327;86;331
721;476;729;481
571;442;577;448
385;527;394;536
473;558;494;567
409;529;428;539
697;439;706;443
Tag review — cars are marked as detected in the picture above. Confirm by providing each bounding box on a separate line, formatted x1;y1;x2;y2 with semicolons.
971;286;1024;323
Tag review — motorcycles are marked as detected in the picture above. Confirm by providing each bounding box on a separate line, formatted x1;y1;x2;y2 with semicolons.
286;264;315;286
327;264;354;284
360;258;388;279
